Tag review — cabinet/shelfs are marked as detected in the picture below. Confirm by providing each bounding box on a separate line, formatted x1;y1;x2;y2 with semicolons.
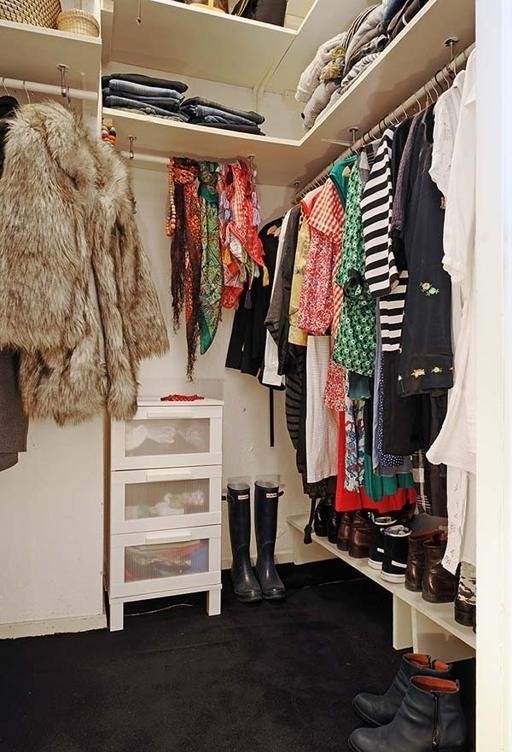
109;395;225;633
1;0;475;187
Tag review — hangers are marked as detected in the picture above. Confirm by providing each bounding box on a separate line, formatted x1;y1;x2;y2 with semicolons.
0;75;73;112
295;53;462;205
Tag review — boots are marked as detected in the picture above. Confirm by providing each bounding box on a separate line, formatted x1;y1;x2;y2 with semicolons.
351;653;453;729
227;483;263;602
347;675;468;752
254;481;287;600
314;497;476;635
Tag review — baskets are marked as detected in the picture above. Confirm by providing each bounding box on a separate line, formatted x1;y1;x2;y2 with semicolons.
55;9;99;38
0;0;62;30
